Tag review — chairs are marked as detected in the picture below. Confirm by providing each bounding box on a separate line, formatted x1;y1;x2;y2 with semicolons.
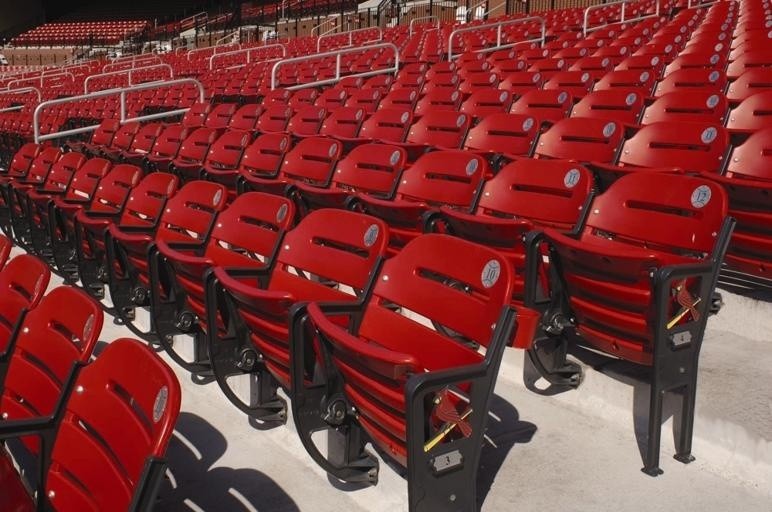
1;0;771;512
